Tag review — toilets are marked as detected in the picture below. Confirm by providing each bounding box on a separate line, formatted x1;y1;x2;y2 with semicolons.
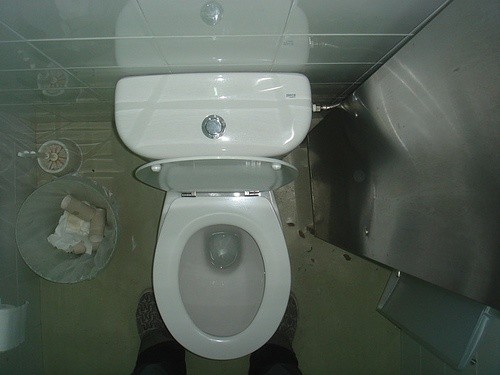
114;72;312;362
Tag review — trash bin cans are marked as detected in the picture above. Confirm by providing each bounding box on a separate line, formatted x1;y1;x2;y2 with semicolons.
15;180;117;284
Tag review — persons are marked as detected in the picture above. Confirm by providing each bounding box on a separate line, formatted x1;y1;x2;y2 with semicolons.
130;289;304;375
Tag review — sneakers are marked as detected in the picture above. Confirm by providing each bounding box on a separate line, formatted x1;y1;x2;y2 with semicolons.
273;291;299;345
135;289;167;336
